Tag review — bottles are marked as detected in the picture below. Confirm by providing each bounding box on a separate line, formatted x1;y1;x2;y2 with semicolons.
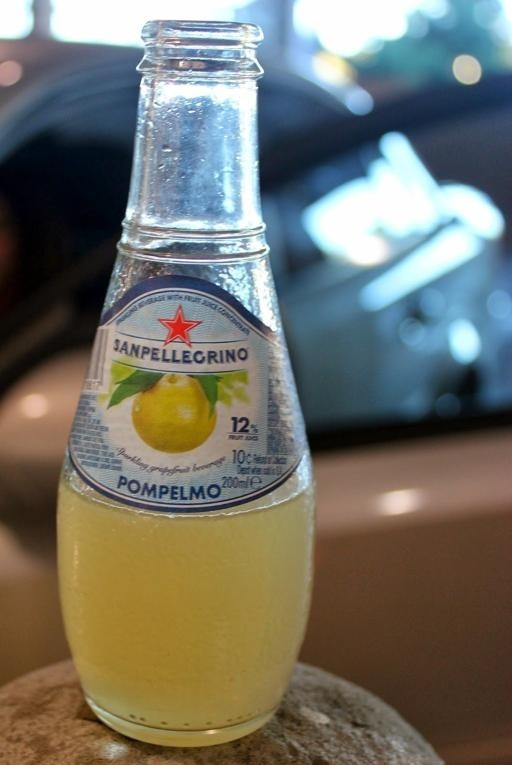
53;14;319;750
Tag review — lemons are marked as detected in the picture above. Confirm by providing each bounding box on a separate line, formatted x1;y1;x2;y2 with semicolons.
132;376;216;454
